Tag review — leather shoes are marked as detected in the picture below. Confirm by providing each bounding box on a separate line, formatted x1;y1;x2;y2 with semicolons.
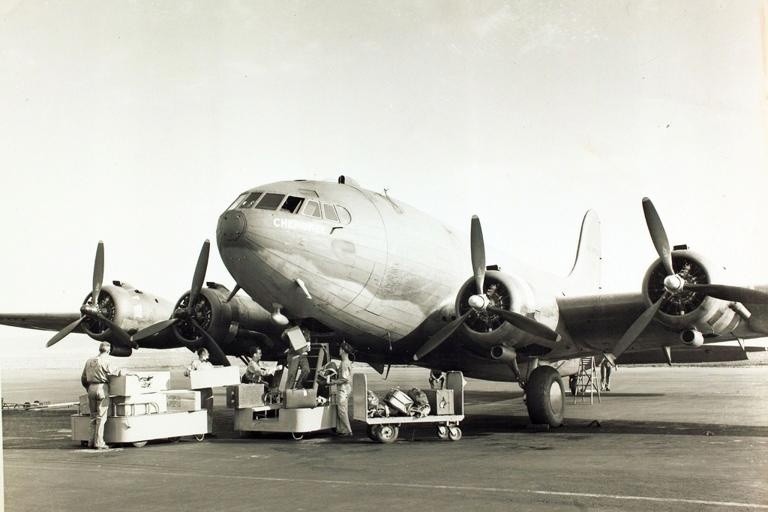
204;433;215;439
88;444;109;450
338;432;354;437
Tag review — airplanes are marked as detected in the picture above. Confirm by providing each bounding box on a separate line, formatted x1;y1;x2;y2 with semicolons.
0;175;768;430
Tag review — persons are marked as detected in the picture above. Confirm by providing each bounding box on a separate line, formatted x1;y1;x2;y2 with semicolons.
316;340;357;436
429;368;444;389
569;375;578;396
281;318;311;390
184;347;216;436
242;344;273;385
80;341;125;450
600;362;617;391
